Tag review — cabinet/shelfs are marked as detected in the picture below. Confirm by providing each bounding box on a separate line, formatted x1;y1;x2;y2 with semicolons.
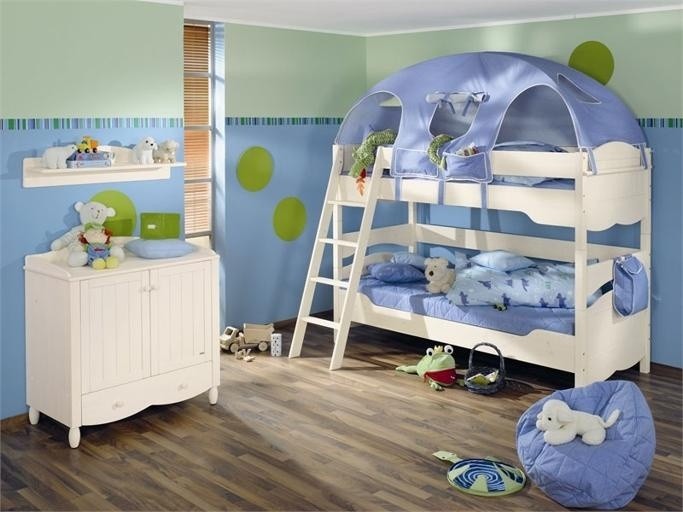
24;235;220;448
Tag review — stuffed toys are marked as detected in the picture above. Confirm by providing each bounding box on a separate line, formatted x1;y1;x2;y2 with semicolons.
392;344;465;391
348;128;454;196
419;256;456;294
39;133;181;270
535;399;620;446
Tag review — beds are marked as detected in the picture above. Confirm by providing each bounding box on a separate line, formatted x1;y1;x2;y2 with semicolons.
287;141;651;389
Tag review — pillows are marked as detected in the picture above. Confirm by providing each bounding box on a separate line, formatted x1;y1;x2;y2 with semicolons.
123;238;200;260
366;249;535;284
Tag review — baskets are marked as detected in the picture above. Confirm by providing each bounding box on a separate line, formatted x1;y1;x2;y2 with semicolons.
464;342;507;396
